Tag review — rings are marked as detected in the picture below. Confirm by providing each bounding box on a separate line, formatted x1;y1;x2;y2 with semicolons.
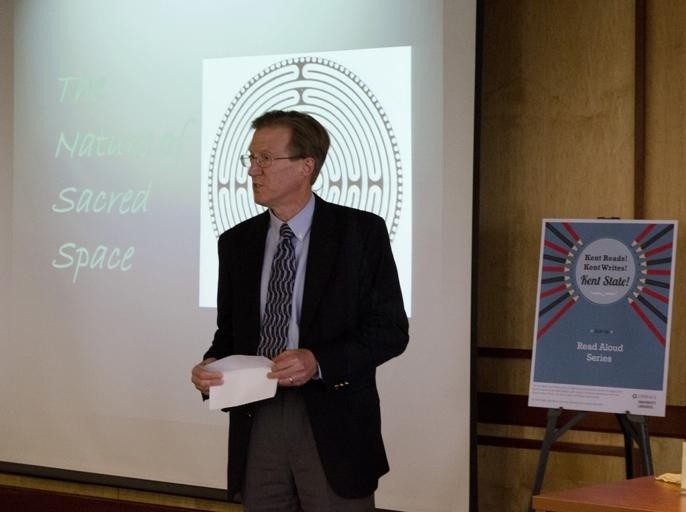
288;376;293;383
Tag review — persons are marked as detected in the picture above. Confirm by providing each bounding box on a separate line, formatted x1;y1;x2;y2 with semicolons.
191;110;410;512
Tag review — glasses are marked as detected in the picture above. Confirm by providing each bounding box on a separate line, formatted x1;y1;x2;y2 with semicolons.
239;150;308;168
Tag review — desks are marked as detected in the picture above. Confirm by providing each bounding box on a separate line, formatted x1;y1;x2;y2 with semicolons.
523;466;686;511
